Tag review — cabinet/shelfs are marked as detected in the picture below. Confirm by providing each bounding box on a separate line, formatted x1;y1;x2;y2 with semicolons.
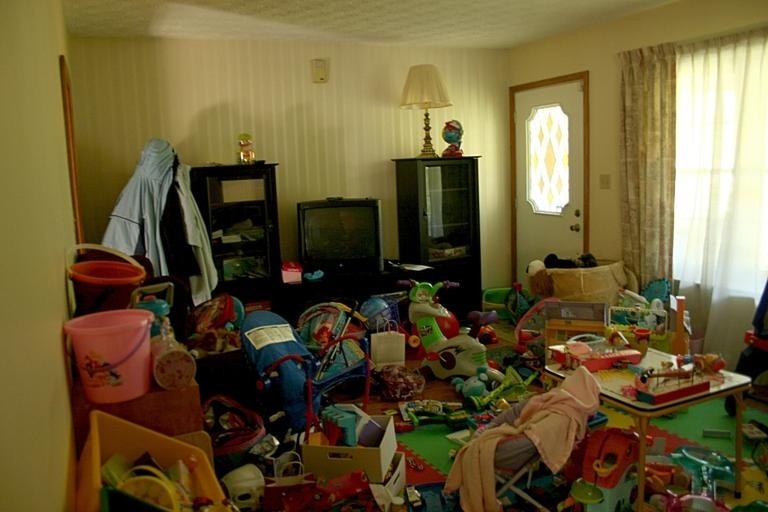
188;162;283;315
392;153;483;327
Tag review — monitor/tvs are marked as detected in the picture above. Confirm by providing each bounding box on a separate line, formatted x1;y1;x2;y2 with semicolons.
297;199;384;272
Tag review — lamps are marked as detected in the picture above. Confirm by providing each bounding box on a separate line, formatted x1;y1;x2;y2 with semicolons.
398;63;453;159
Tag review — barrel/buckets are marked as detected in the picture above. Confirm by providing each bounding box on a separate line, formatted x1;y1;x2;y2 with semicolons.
62;307;156;403
65;243;146;314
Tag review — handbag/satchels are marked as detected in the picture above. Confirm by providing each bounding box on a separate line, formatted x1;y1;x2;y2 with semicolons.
262;450;317;512
370;317;406;372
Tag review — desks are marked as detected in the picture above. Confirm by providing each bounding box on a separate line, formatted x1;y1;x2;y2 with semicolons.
540;346;754;512
